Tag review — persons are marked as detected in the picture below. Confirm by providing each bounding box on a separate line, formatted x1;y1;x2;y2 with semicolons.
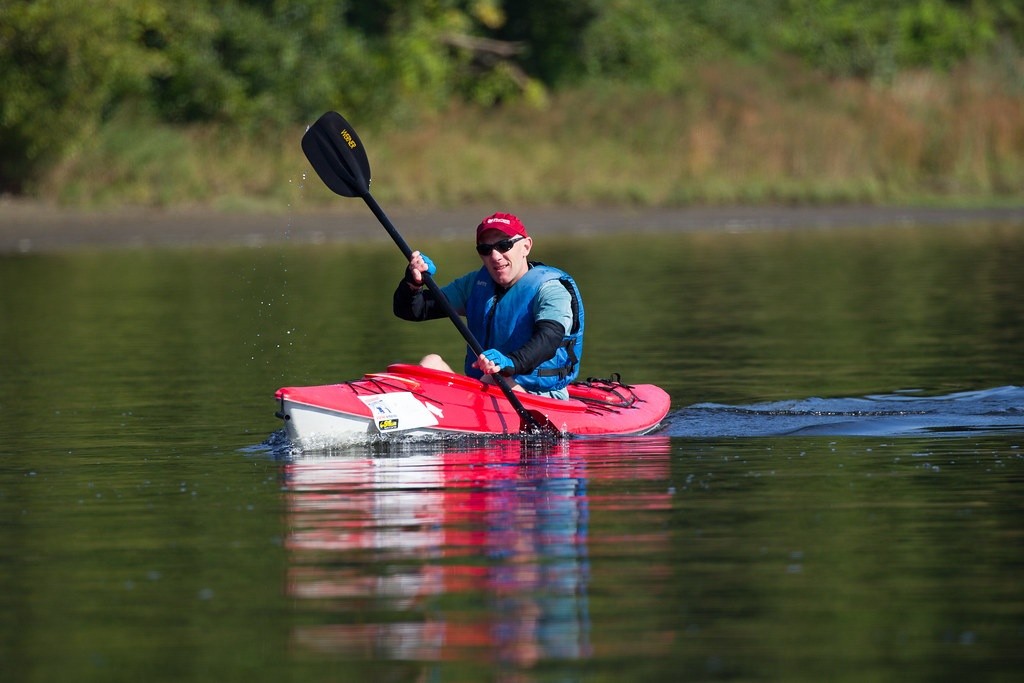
393;213;585;400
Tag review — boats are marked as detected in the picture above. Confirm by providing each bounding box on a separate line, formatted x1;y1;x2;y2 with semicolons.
274;361;672;452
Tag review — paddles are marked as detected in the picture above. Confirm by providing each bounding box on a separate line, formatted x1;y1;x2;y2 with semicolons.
299;110;564;439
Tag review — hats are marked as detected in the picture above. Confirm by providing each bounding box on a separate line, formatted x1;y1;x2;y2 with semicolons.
476;212;528;245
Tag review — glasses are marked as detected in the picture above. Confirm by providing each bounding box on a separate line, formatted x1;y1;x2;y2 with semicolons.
476;236;527;256
